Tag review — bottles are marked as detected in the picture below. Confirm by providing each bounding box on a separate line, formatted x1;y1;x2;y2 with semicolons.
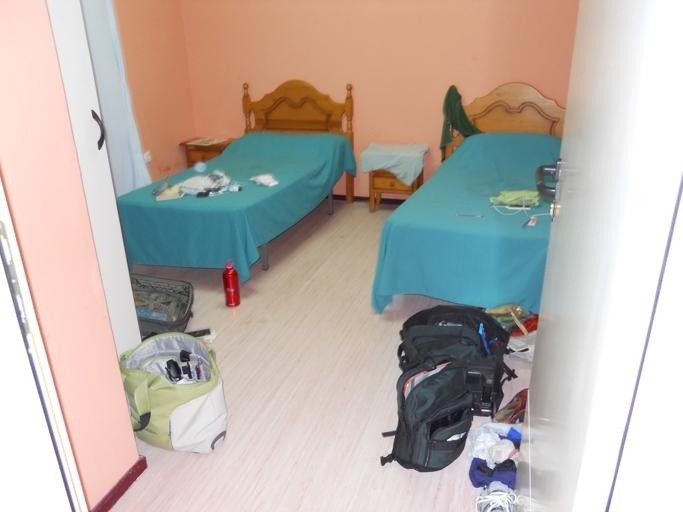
221;261;241;308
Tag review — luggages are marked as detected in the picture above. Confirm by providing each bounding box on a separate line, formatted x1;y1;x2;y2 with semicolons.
130;273;194;336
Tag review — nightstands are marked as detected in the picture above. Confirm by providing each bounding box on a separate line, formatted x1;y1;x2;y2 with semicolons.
180;136;233;169
367;143;425;212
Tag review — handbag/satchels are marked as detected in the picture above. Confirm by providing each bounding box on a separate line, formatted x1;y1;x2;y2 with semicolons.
119;328;227;455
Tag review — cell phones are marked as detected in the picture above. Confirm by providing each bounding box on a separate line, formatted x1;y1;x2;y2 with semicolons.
507;205;531;210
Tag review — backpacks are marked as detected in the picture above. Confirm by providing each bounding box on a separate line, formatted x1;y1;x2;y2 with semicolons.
380;305;529;472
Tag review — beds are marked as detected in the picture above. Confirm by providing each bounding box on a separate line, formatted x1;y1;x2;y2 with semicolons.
372;80;566;315
115;80;355;285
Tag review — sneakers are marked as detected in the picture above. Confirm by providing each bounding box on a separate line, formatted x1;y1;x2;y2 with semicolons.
476;481;517;512
